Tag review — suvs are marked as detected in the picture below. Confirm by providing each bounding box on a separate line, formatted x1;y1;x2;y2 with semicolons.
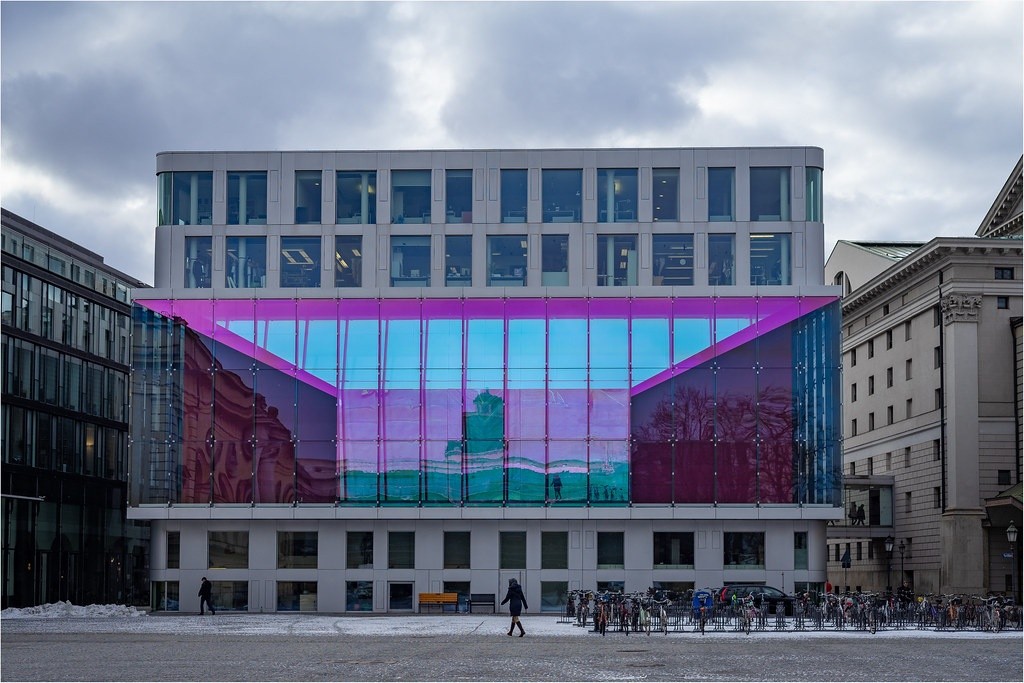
715;585;799;615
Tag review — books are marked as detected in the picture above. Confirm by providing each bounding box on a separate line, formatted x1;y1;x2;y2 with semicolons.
1007;599;1013;603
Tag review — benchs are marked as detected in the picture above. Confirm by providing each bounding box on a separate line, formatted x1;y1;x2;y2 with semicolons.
469;594;496;613
418;593;459;613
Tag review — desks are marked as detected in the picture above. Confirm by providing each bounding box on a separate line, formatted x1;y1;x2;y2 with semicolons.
300;594;316;611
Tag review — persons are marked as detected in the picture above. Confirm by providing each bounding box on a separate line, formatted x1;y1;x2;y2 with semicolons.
501;578;528;637
122;574;136;601
988;591;1006;605
550;475;563;499
848;502;866;525
897;581;914;603
197;577;215;615
826;580;832;593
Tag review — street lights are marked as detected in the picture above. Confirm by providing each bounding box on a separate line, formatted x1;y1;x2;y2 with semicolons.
1006;520;1019;605
884;534;894;595
899;541;907;596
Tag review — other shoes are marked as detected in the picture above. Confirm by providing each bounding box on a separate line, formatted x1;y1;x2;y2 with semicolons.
211;611;215;615
197;612;204;615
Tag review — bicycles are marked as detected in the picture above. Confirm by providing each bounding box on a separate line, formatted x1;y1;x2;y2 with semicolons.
564;589;1022;637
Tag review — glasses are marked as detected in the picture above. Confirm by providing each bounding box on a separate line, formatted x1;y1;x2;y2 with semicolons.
904;582;908;584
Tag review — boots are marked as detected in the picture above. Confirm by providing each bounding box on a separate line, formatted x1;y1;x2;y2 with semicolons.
516;621;525;637
507;622;515;636
